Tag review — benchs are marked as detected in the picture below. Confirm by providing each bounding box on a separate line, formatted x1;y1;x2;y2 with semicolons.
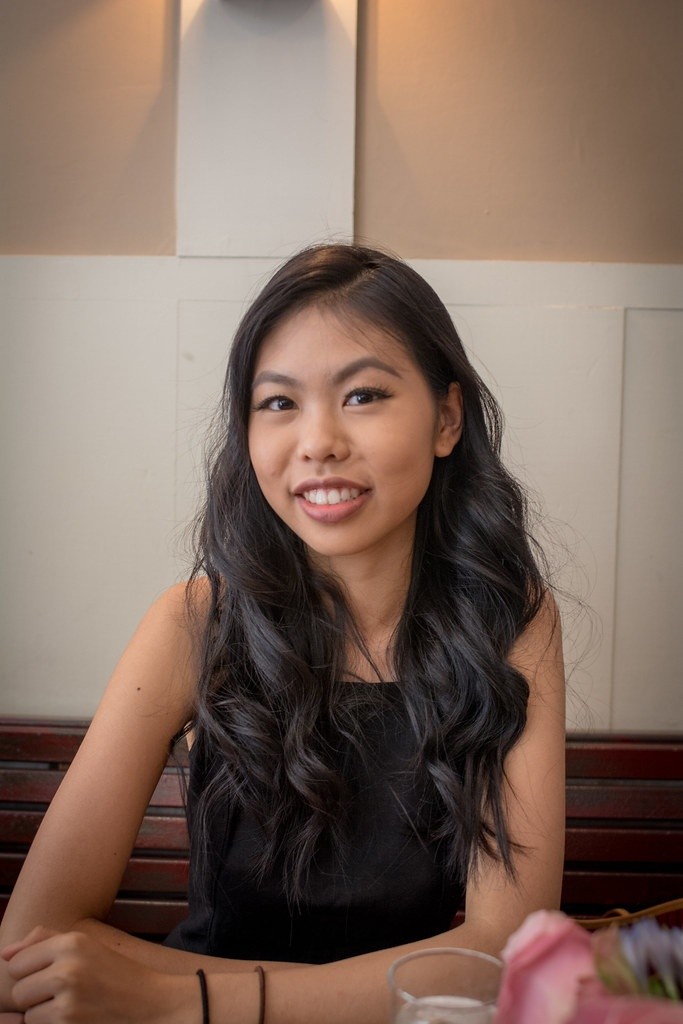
0;716;683;950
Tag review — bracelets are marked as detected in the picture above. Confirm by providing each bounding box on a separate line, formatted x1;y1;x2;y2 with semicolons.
196;969;210;1024
254;966;265;1024
1;241;567;1024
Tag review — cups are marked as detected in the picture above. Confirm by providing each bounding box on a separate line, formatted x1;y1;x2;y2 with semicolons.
386;946;504;1024
